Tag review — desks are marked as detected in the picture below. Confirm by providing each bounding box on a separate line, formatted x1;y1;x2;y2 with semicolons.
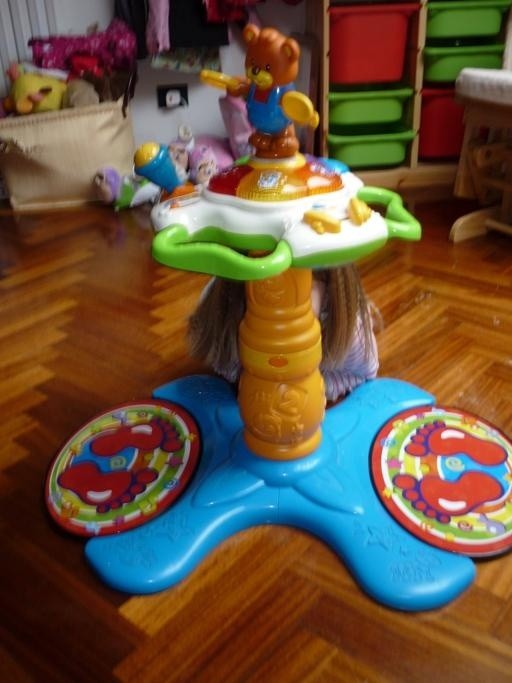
448;67;511;243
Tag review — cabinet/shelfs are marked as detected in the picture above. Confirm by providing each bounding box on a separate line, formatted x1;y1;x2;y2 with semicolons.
307;0;511;191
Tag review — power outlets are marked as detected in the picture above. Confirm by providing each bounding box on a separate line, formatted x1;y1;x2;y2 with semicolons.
157;85;188;108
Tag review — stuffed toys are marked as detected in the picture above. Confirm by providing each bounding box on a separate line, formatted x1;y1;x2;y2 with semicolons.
166;121;196;171
3;61;68;116
88;166;162;214
185;145;221;189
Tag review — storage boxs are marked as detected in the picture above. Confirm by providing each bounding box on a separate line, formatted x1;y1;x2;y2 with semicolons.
426;0;512;38
419;88;491;158
326;129;415;168
327;86;415;126
423;45;505;84
327;2;421;83
